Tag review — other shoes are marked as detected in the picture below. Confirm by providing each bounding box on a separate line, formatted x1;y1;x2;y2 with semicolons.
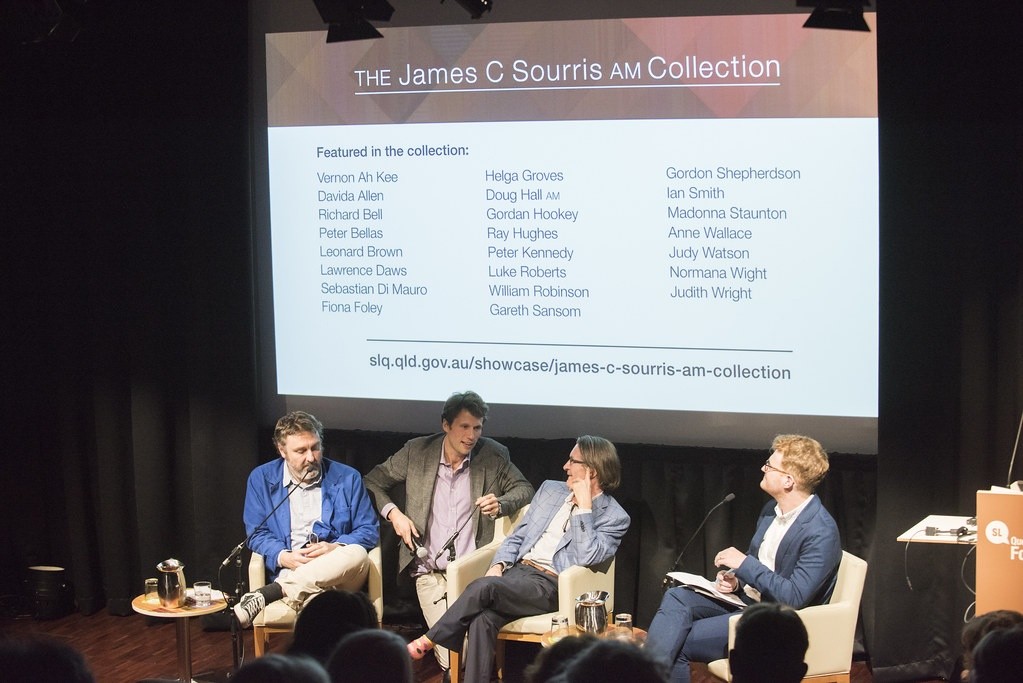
408;652;422;674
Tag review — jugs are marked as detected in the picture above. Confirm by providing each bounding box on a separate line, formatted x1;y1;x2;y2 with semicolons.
574;590;610;637
157;558;187;609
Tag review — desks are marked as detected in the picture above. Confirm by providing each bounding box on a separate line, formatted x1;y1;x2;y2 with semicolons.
131;591;230;683
538;624;652;655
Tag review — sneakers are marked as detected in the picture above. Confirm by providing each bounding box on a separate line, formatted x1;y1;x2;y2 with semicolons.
234;590;266;629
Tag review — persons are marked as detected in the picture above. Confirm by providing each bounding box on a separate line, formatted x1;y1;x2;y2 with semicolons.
403;433;632;683
1;626;96;683
230;408;380;656
325;629;414;683
286;588;381;675
229;651;334;683
728;603;810;683
361;392;536;683
960;609;1023;683
637;434;842;683
519;630;670;682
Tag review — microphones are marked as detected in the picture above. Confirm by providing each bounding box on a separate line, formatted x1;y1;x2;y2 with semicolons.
224;464;314;566
663;492;734;583
435;449;507;557
411;536;429;558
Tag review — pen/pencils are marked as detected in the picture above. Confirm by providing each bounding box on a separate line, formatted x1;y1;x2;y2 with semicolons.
715;568;734;583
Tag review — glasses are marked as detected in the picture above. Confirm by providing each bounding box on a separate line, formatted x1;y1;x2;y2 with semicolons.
309;532;322;544
570;456;584;465
765;460;794;484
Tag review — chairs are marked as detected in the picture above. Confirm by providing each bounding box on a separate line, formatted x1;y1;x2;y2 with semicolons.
699;551;868;683
245;531;384;662
443;495;618;683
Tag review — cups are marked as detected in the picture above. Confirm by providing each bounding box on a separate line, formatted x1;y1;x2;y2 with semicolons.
193;581;211;607
551;616;569;642
144;578;159;604
615;613;633;642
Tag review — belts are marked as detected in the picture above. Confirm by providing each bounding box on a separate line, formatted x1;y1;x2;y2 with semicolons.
522;559;557;577
429;569;446;574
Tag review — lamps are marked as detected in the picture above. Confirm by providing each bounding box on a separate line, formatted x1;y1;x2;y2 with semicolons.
794;0;875;36
310;0;396;47
